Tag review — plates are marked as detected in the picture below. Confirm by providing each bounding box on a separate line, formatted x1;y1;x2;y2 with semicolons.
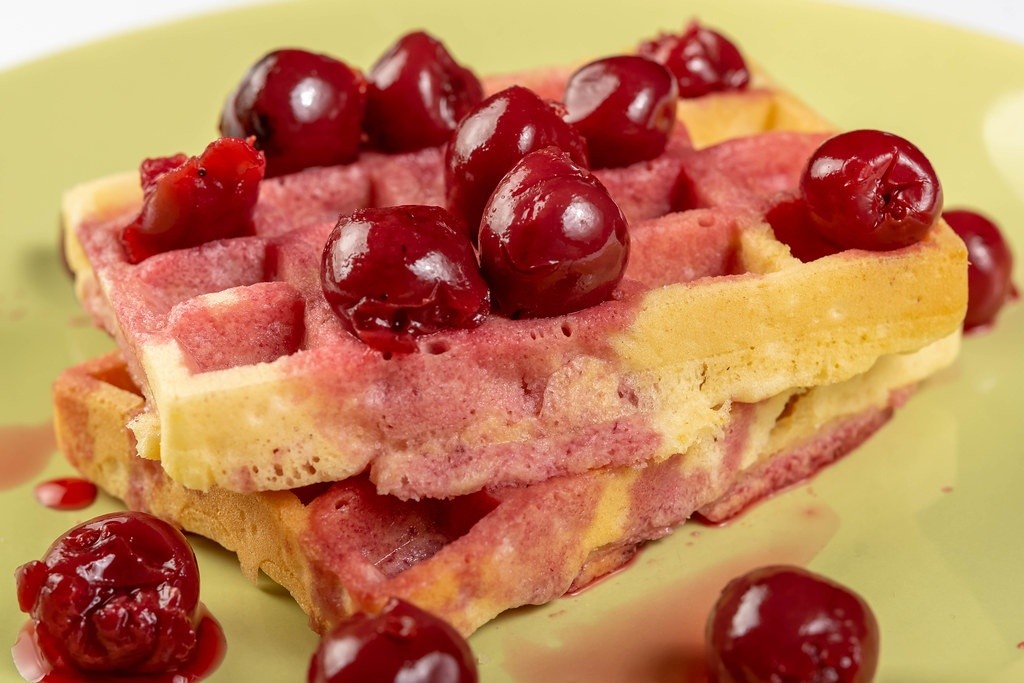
2;2;1024;683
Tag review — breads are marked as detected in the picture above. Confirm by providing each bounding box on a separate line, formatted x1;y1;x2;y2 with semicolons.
47;20;967;636
